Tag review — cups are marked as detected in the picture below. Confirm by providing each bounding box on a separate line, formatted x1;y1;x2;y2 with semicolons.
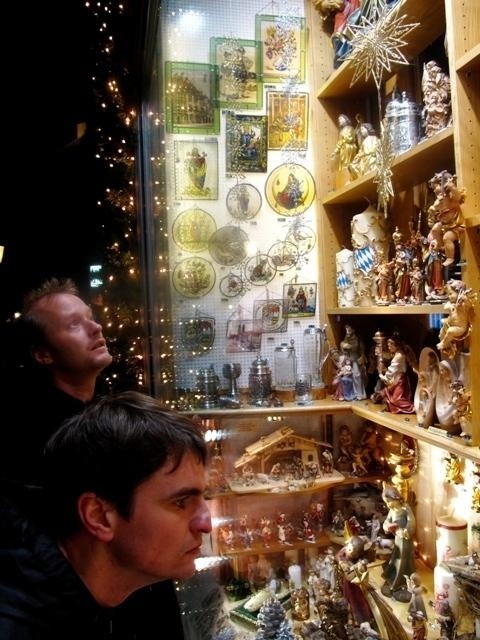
295;374;313;405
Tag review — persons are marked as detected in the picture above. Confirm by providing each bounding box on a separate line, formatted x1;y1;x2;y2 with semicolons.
1;282;125;473
332;116;357;186
321;325;368;400
220;501;349;552
0;392;212;640
427;171;467;266
370;332;412;413
436;279;477;359
381;486;415;600
225;419;389;493
351;124;383;175
372;252;395;304
393;211;447;305
400;573;427;624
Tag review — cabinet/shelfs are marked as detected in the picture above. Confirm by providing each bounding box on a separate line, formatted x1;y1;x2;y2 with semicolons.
172;0;478;640
